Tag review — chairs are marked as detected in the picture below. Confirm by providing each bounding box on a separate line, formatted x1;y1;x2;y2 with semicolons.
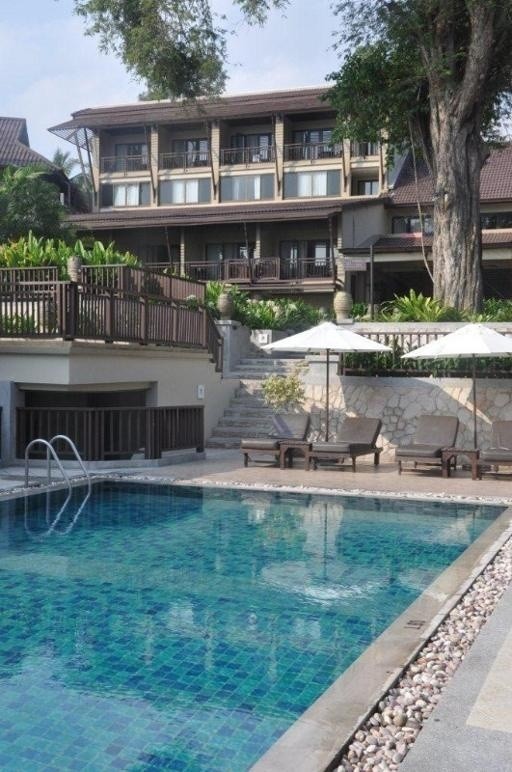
240;412;311;470
310;416;384;472
479;419;512;479
394;414;461;477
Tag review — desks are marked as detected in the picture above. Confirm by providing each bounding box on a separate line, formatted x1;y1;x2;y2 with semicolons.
440;446;481;480
278;439;314;472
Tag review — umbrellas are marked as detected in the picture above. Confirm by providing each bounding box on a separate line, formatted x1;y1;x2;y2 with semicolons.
262;499;387;603
260;320;393;441
401;506;477;602
400;317;512;450
